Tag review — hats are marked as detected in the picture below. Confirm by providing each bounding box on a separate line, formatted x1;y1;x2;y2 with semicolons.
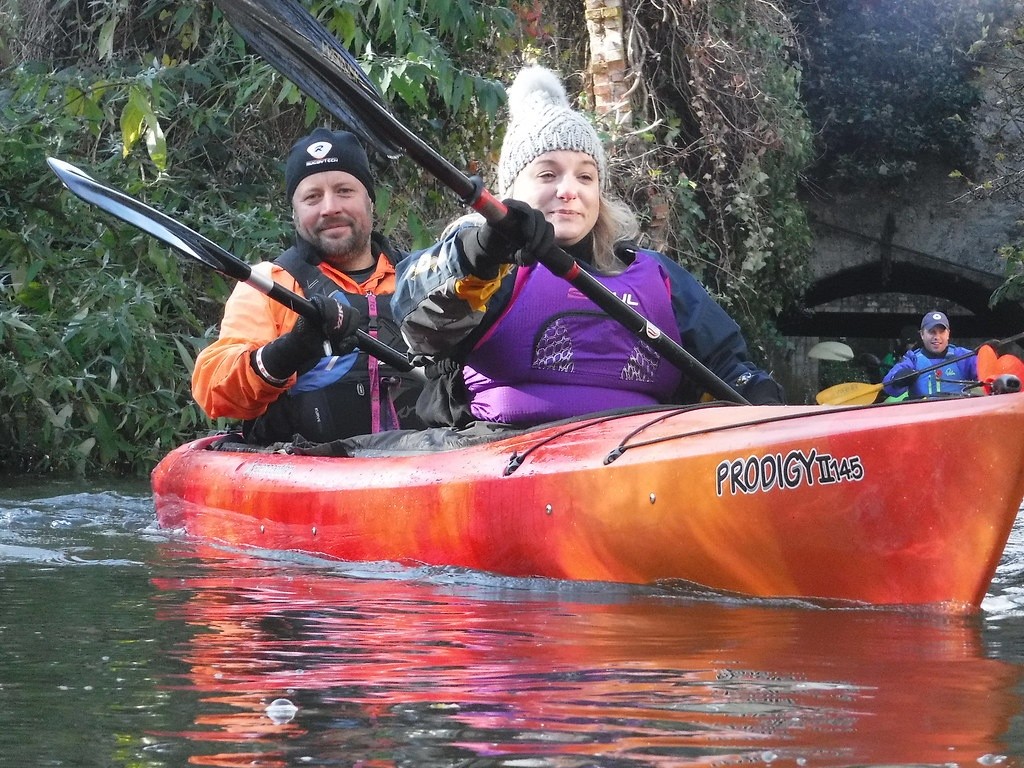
921;311;950;330
286;125;375;207
498;65;606;194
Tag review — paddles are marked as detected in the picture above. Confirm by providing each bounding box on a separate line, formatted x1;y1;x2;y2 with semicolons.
807;340;894;371
47;157;428;380
210;1;754;404
813;332;1024;407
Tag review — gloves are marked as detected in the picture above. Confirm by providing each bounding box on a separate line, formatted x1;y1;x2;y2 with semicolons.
893;370;920;388
746;378;783;407
276;295;362;369
478;199;556;269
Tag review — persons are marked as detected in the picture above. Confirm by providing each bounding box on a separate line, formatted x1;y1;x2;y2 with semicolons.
191;130;428;446
389;59;786;425
882;311;978;397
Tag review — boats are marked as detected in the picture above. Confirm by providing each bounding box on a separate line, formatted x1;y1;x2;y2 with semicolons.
147;387;1024;619
